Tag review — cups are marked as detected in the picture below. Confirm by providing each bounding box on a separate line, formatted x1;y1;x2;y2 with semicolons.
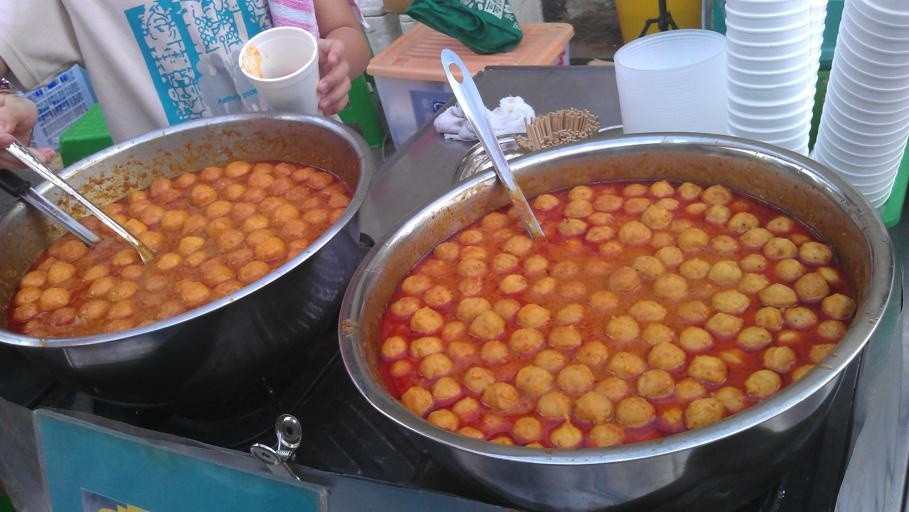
725;1;909;214
611;27;726;134
237;25;320;117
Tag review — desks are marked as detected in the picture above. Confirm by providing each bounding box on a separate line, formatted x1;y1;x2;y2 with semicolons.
0;65;908;512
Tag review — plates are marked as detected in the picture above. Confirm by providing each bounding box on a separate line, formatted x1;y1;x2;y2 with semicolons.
450;133;526;187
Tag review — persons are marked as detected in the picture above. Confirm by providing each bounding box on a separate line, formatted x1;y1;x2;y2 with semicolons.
1;0;372;170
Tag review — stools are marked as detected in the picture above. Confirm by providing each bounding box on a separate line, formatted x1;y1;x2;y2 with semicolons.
59;101;115;170
336;72;386;149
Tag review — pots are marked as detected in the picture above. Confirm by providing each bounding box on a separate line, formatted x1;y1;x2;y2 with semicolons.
0;110;375;407
338;132;898;511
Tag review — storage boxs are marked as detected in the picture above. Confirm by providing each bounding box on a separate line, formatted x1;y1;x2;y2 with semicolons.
363;19;577;154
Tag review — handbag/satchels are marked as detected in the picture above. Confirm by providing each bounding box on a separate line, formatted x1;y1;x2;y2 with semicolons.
404;0;522;55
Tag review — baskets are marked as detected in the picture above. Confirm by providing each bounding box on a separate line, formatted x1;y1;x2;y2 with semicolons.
16;64;97;151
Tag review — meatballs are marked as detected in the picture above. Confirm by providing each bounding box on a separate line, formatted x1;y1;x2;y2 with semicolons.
378;180;856;450
12;160;350;339
